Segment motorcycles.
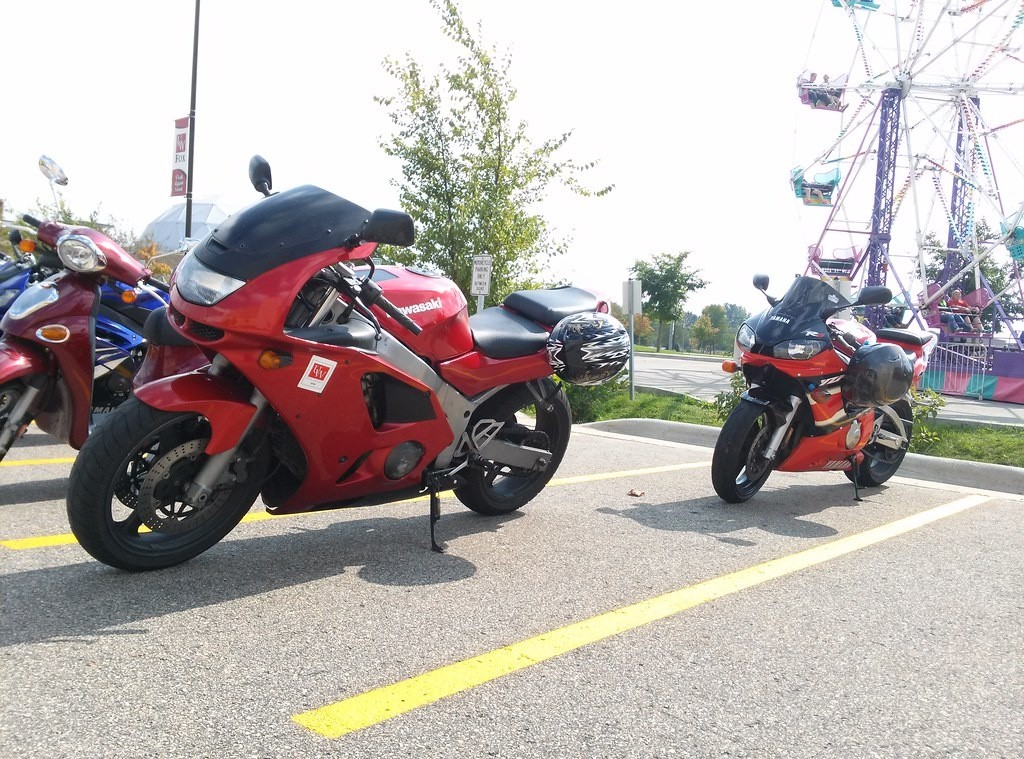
[0,155,202,465]
[67,154,631,574]
[710,273,942,505]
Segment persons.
[947,290,991,333]
[938,294,978,334]
[872,304,909,329]
[802,73,849,112]
[794,168,829,205]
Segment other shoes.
[969,328,977,333]
[983,329,991,333]
[822,201,829,204]
[806,198,812,204]
[953,328,963,333]
[816,98,850,111]
[896,324,908,329]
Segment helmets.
[546,312,631,386]
[842,344,914,406]
